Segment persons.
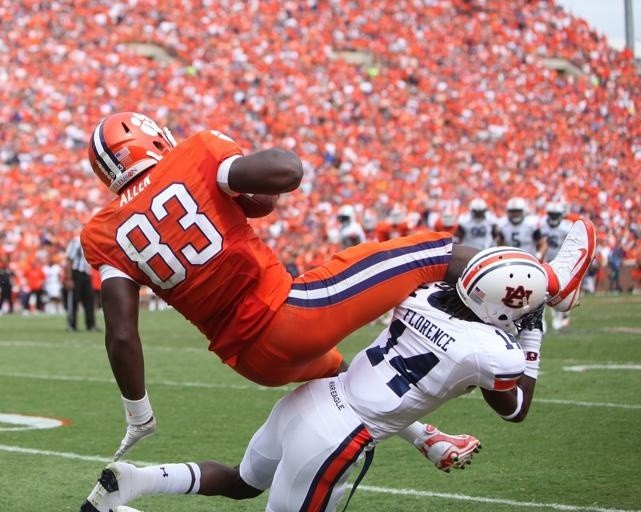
[79,245,549,512]
[81,112,596,473]
[1,0,640,330]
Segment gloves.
[111,420,158,460]
[514,311,544,347]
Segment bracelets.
[516,327,541,382]
[121,388,154,425]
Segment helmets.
[457,246,548,336]
[506,198,526,224]
[470,199,488,218]
[547,204,563,226]
[88,113,175,193]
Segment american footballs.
[235,193,279,218]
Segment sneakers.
[80,461,135,511]
[412,423,480,472]
[548,219,596,312]
